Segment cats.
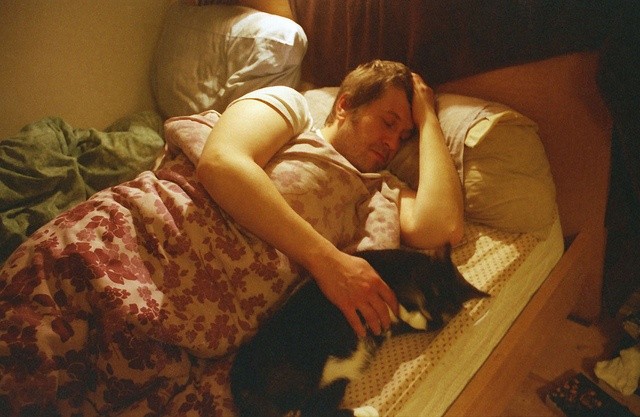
[230,241,492,417]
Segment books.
[544,372,640,417]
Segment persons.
[2,58,466,417]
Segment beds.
[1,51,612,417]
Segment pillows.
[149,1,307,118]
[293,88,556,233]
